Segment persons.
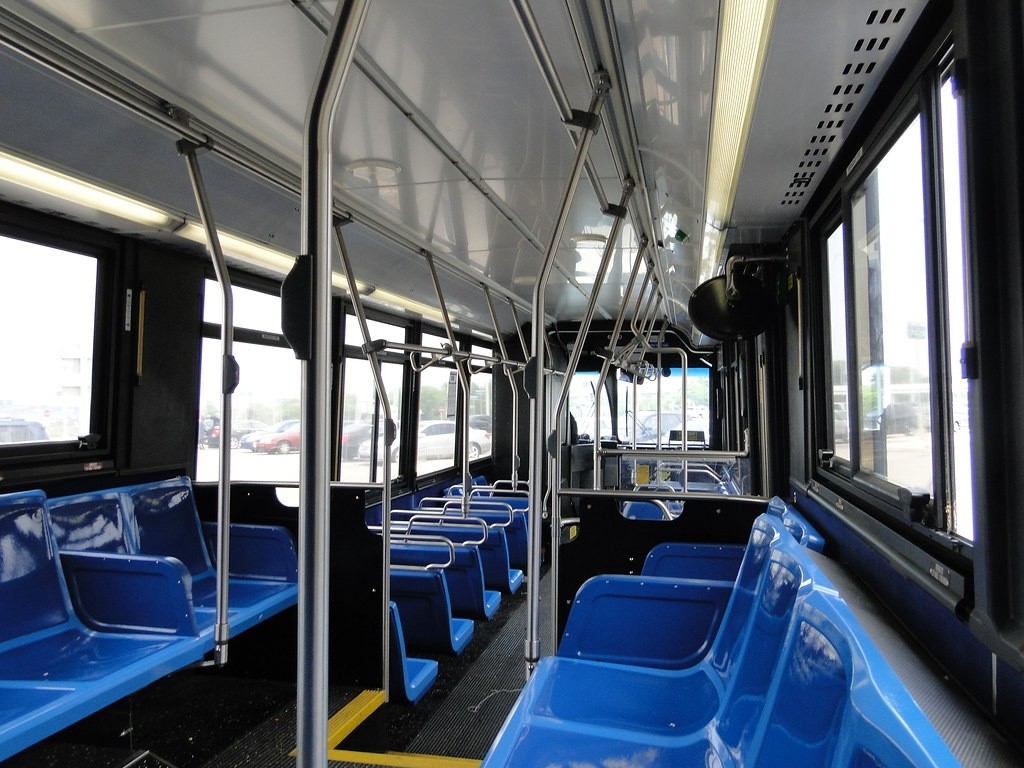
[619,368,630,382]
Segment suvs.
[0,417,50,445]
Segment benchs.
[0,462,1024,768]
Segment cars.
[619,406,704,446]
[198,413,492,464]
[834,397,971,443]
[378,419,492,464]
